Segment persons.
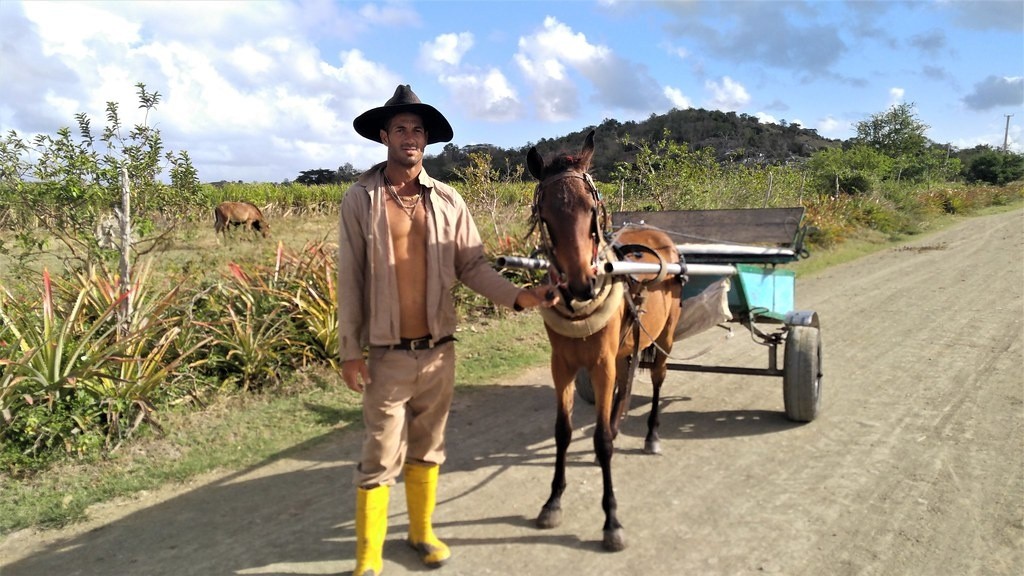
[337,85,561,576]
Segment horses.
[528,129,683,551]
[213,201,271,240]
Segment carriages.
[486,132,824,554]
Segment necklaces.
[383,169,426,221]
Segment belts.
[382,337,459,350]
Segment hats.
[353,85,453,145]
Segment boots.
[353,486,389,576]
[402,462,451,568]
